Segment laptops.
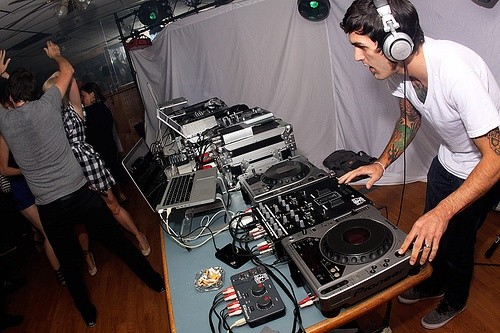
[157,109,217,139]
[122,137,217,214]
[146,82,187,110]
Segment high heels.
[86,253,97,276]
[138,234,151,256]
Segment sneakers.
[421,302,468,330]
[397,287,445,303]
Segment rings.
[424,243,431,248]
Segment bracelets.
[373,162,385,176]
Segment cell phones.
[215,244,253,269]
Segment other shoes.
[158,275,166,294]
[54,267,67,287]
[86,304,96,328]
[0,314,24,329]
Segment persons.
[79,81,127,203]
[1,71,151,277]
[0,39,165,328]
[1,91,68,286]
[336,0,500,329]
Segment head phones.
[372,0,415,62]
